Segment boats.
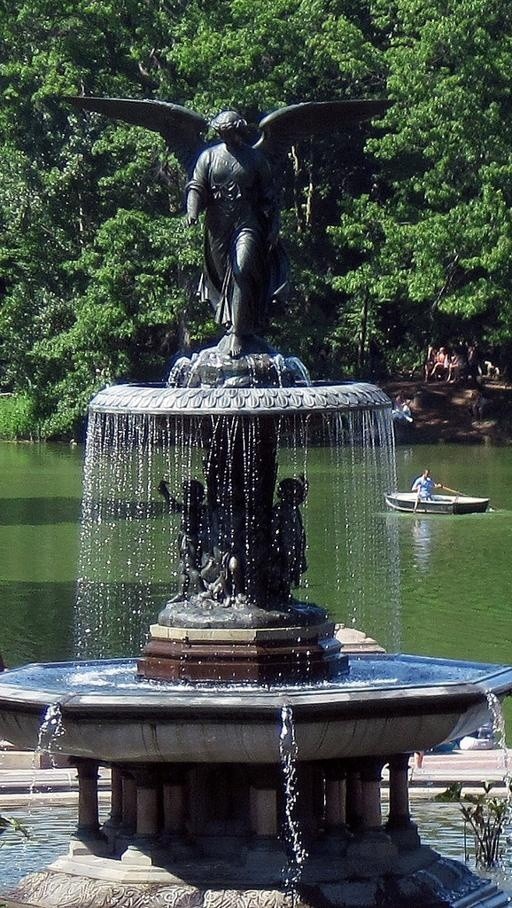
[387,510,509,523]
[384,491,490,516]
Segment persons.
[276,475,308,600]
[185,109,283,350]
[209,480,242,608]
[395,390,411,416]
[411,468,442,501]
[159,476,206,603]
[425,339,476,381]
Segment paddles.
[441,485,498,511]
[413,487,421,514]
